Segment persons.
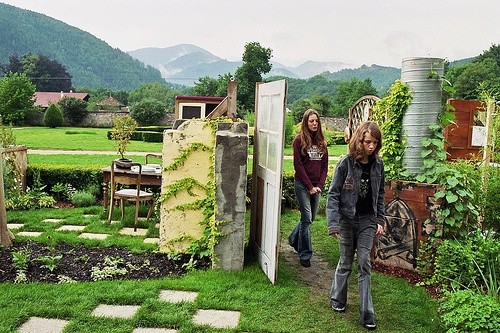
[325,122,386,329]
[287,109,329,268]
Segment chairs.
[143,152,163,208]
[106,159,156,231]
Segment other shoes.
[300,256,310,267]
[362,319,376,328]
[291,240,298,252]
[331,301,345,311]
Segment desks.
[100,165,162,215]
